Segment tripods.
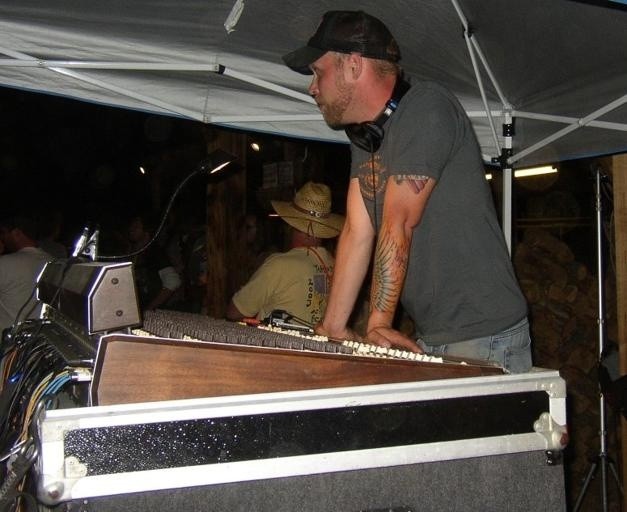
[568,170,624,512]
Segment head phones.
[345,77,412,153]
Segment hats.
[272,182,346,239]
[282,10,401,75]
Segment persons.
[224,180,347,332]
[280,11,534,374]
[0,204,281,335]
